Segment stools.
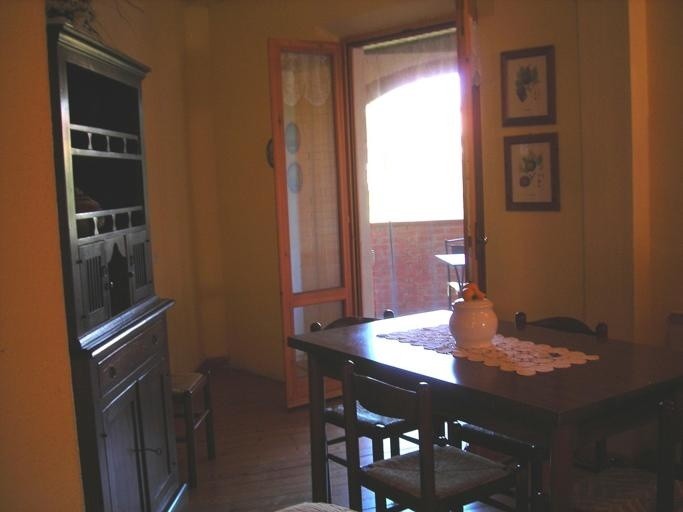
[171,372,215,489]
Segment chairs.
[631,310,682,510]
[448,312,606,512]
[341,358,522,511]
[445,238,466,310]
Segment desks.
[434,253,465,291]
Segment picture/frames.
[503,133,561,211]
[500,46,556,127]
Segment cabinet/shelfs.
[47,23,188,512]
